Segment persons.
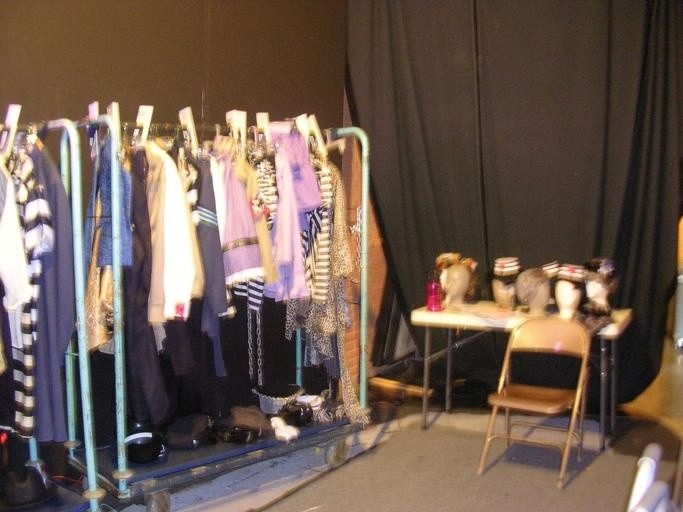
[434,251,621,324]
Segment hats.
[0,467,56,512]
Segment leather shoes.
[268,403,313,427]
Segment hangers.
[26,120,44,150]
[119,121,329,162]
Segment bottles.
[426,279,441,311]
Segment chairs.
[477,317,591,490]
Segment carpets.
[246,418,683,512]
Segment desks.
[411,301,634,452]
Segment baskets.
[252,383,304,414]
